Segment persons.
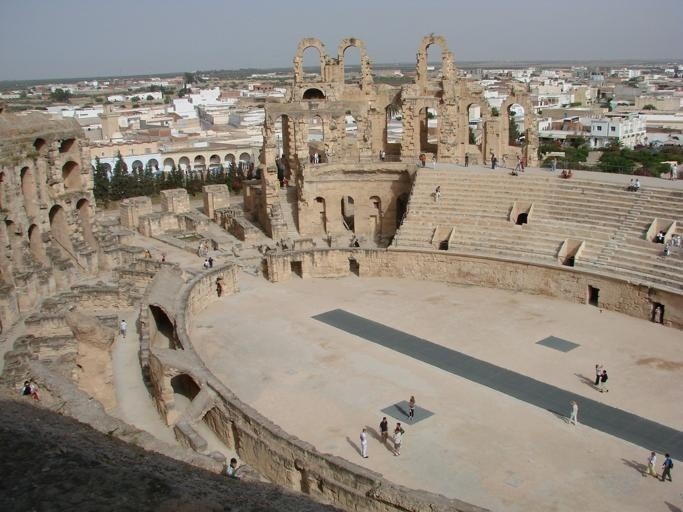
[627,178,634,192]
[431,158,436,169]
[656,230,682,257]
[119,319,128,339]
[281,177,288,188]
[314,151,319,163]
[381,151,386,161]
[654,307,662,323]
[24,380,39,400]
[670,163,678,183]
[419,154,427,167]
[379,417,388,443]
[566,399,579,427]
[644,451,660,478]
[311,152,316,164]
[593,363,604,386]
[391,429,402,457]
[225,458,241,481]
[408,396,416,420]
[408,399,415,413]
[435,186,442,201]
[20,381,30,396]
[633,178,641,192]
[144,241,214,270]
[395,422,405,449]
[359,428,369,458]
[464,153,470,167]
[598,369,609,392]
[491,153,573,179]
[659,454,674,482]
